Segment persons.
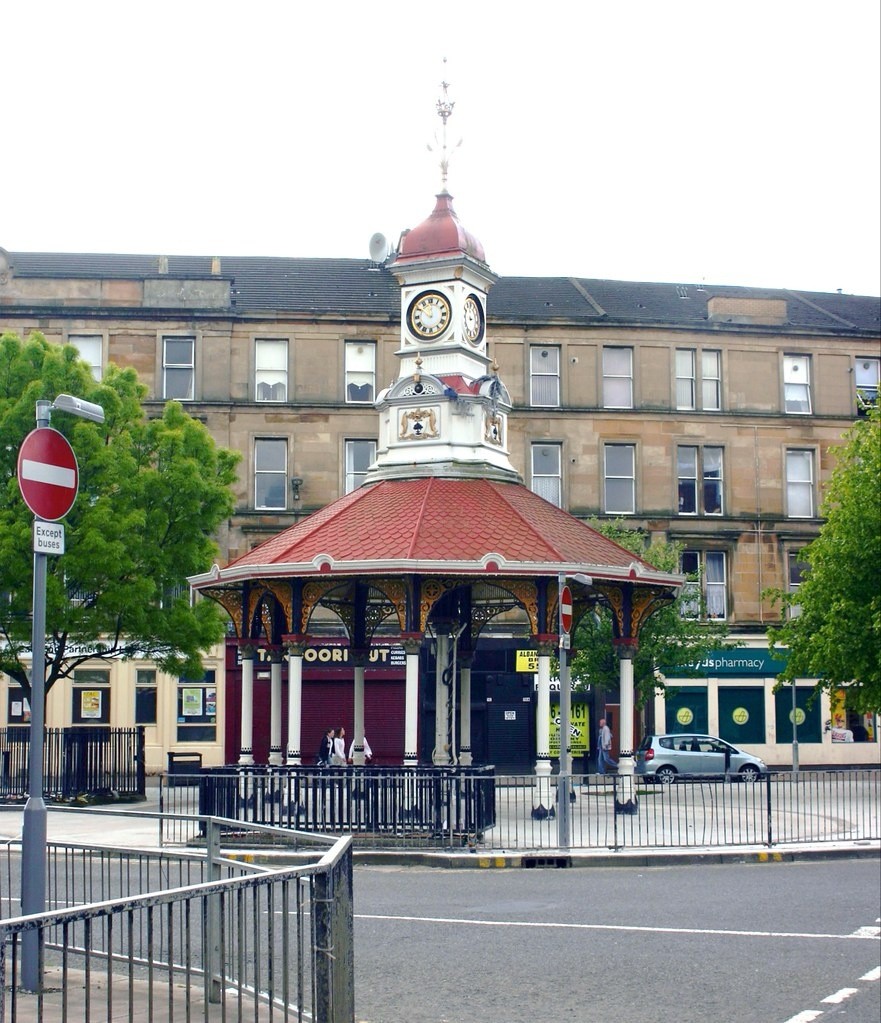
[318,726,335,765]
[597,718,618,775]
[348,728,372,763]
[334,726,347,765]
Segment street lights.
[557,569,593,852]
[22,393,106,993]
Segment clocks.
[410,295,449,337]
[464,300,482,340]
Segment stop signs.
[19,427,79,521]
[561,586,573,633]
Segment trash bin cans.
[167,750,203,786]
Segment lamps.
[292,477,303,500]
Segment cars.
[635,735,769,784]
